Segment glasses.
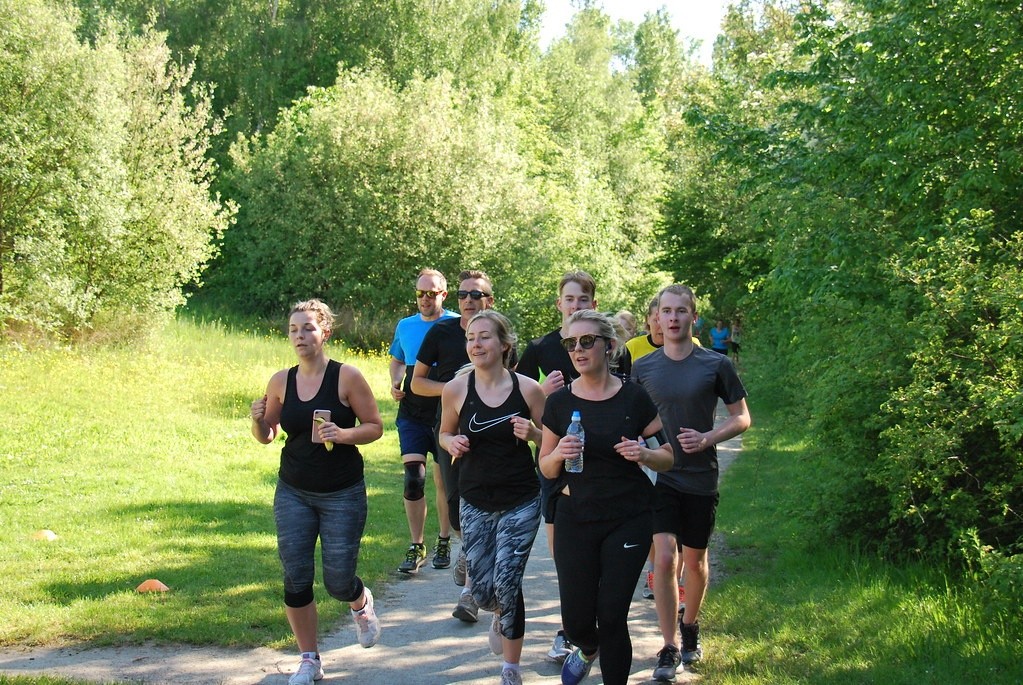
[415,289,444,298]
[457,291,490,300]
[560,334,606,352]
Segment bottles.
[565,411,585,473]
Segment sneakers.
[643,572,654,599]
[431,534,452,569]
[679,614,702,663]
[289,652,323,685]
[678,582,687,613]
[562,648,599,685]
[652,645,684,683]
[501,669,522,685]
[452,548,466,586]
[351,586,381,649]
[396,546,428,574]
[489,613,503,655]
[548,631,574,661]
[452,588,478,623]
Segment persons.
[538,311,674,685]
[632,284,751,682]
[709,319,731,354]
[439,309,545,685]
[692,318,704,339]
[627,297,701,612]
[730,319,742,363]
[251,301,383,685]
[515,269,633,662]
[389,270,462,574]
[614,309,636,337]
[411,271,519,622]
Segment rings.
[631,452,633,455]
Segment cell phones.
[312,410,331,444]
